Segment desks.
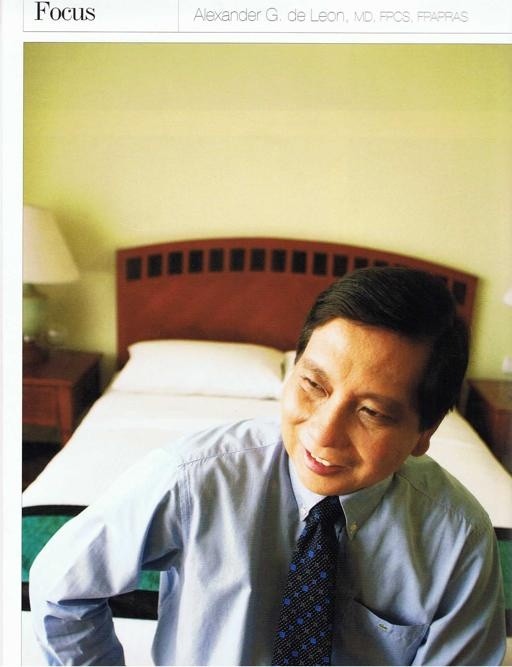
[23,345,101,453]
[464,375,512,474]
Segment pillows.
[114,338,287,398]
[281,349,297,379]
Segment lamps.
[23,203,84,357]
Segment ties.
[272,497,345,665]
